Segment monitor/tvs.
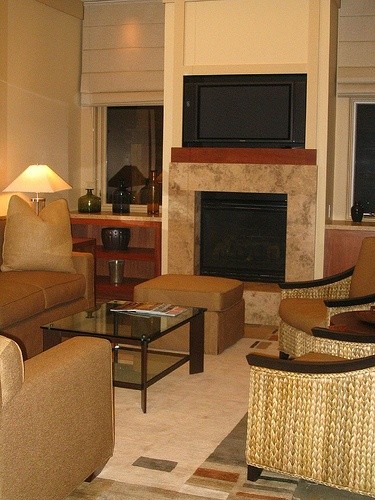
[182,74,307,150]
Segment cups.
[108,258,125,284]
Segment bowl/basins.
[101,228,130,251]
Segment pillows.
[0,195,78,275]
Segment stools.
[134,274,245,355]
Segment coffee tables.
[40,299,207,413]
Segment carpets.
[65,323,301,500]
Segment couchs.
[0,216,95,362]
[277,237,375,359]
[245,327,375,500]
[0,335,115,500]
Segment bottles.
[113,176,130,214]
[78,188,101,214]
[147,170,159,217]
[351,201,363,222]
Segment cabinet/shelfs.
[70,210,161,305]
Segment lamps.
[2,163,73,215]
